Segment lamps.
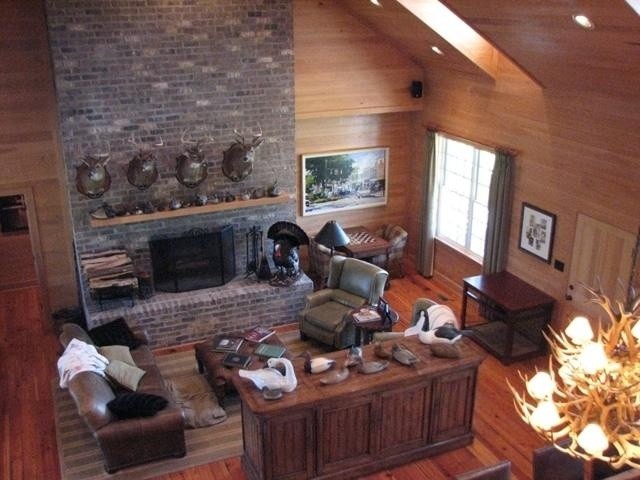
[314,221,352,257]
[505,276,640,471]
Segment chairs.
[298,255,389,351]
[369,223,408,291]
[372,298,454,343]
[583,438,640,480]
[533,438,583,479]
[455,459,512,479]
[308,235,347,291]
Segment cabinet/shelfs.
[231,329,486,480]
[461,271,557,366]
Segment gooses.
[238,357,297,392]
[416,309,462,344]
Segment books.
[245,359,254,368]
[351,308,381,327]
[211,334,245,354]
[238,325,276,344]
[253,343,286,359]
[223,351,251,370]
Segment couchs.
[58,322,186,474]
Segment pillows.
[106,392,169,418]
[99,345,136,366]
[102,359,146,392]
[88,317,140,351]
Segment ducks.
[431,340,468,359]
[294,340,419,384]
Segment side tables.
[351,306,400,347]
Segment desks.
[342,225,393,261]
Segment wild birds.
[267,221,309,280]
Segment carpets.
[50,321,338,480]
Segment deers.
[176,128,214,188]
[127,131,164,190]
[221,122,264,181]
[76,141,111,199]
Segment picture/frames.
[518,202,556,265]
[300,144,390,218]
